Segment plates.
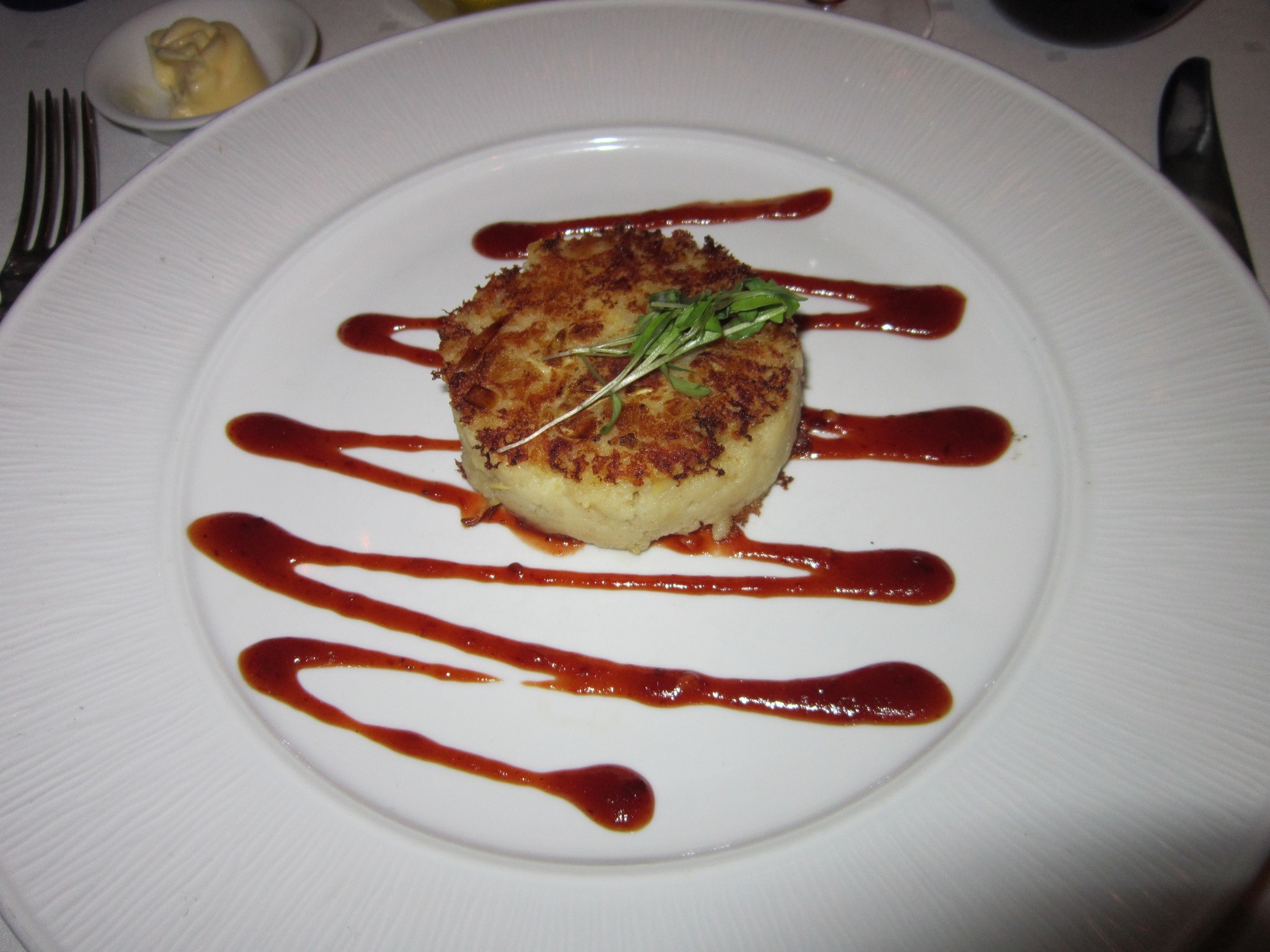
[1,0,1270,952]
[83,0,319,146]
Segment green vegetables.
[496,277,810,455]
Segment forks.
[0,88,95,317]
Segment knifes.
[1157,56,1256,278]
